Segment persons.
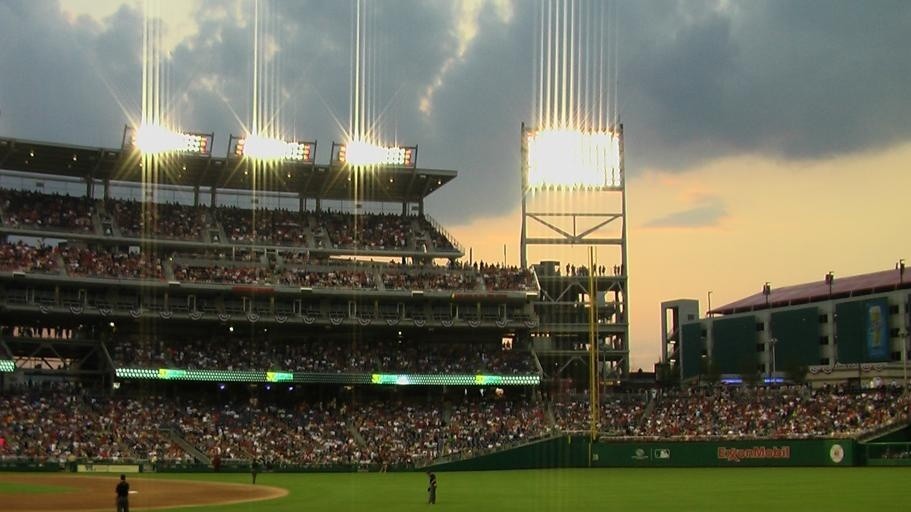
[425,470,437,504]
[249,457,258,484]
[565,263,624,276]
[0,234,536,292]
[0,184,456,253]
[2,383,909,472]
[104,321,542,377]
[115,473,130,512]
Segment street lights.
[225,132,317,210]
[521,122,628,277]
[327,141,419,216]
[117,125,214,207]
